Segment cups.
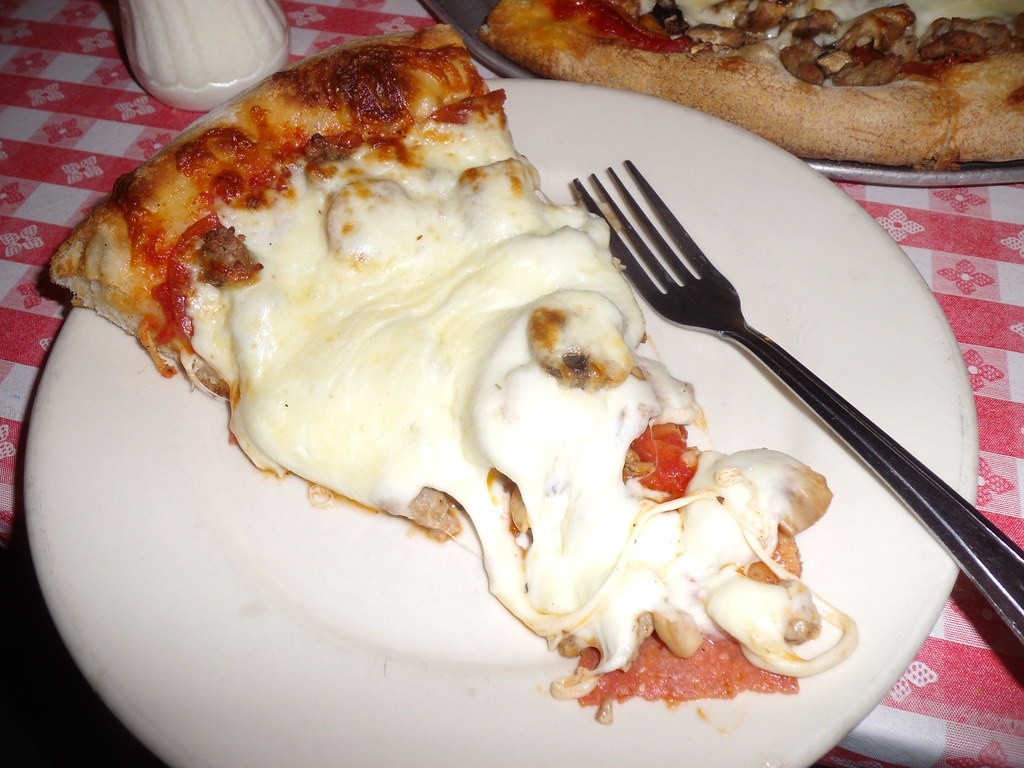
[115,1,290,107]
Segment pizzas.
[50,22,858,696]
[477,0,1022,165]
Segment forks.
[571,158,1024,649]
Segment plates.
[23,78,977,768]
